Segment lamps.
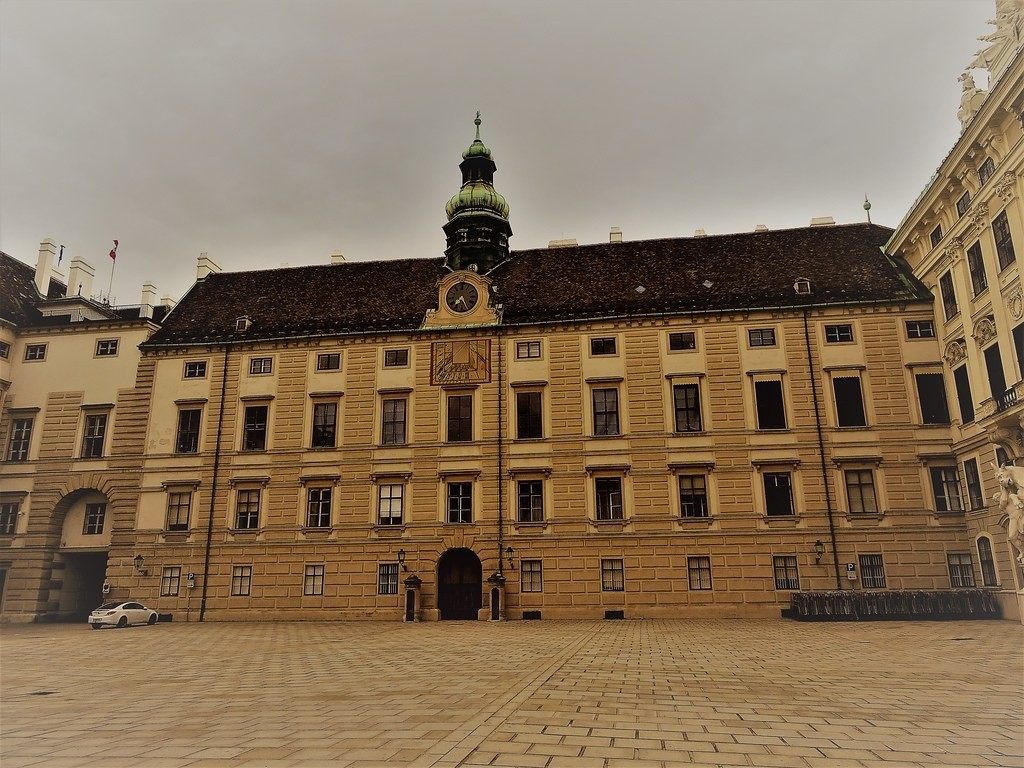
[814,539,825,561]
[398,549,407,570]
[133,554,148,576]
[505,547,514,568]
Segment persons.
[992,492,1024,562]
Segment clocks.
[446,280,480,313]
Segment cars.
[88,601,159,629]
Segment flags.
[110,239,118,260]
